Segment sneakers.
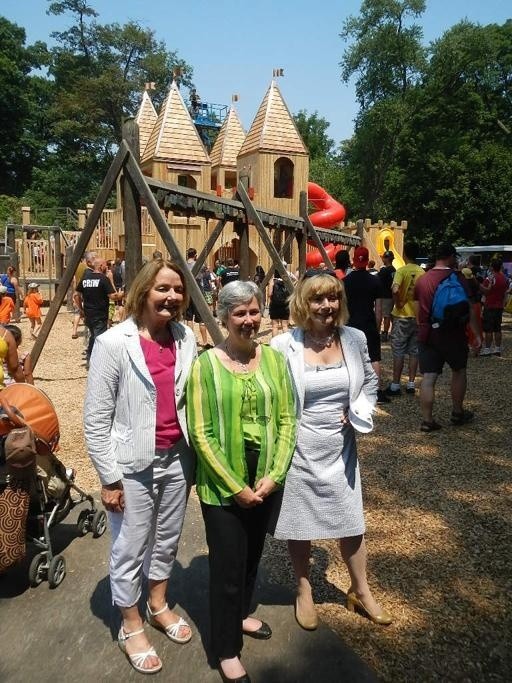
[203,343,214,349]
[381,333,392,344]
[30,327,38,340]
[72,334,78,338]
[470,348,501,360]
[376,383,415,404]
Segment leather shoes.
[242,616,272,639]
[215,656,252,683]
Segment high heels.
[347,587,393,624]
[295,594,318,629]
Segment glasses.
[303,268,336,280]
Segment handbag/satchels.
[202,289,214,305]
[503,292,512,313]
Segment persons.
[153,251,162,259]
[333,240,509,432]
[0,228,61,486]
[72,248,126,368]
[269,268,392,630]
[82,258,198,673]
[184,248,239,350]
[254,261,298,337]
[185,280,298,682]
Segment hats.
[5,425,37,481]
[380,251,394,259]
[335,250,351,269]
[352,246,368,267]
[434,242,461,256]
[27,283,40,289]
[461,268,473,279]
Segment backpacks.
[273,280,287,308]
[429,273,470,331]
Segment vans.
[453,245,512,276]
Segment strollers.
[1,382,107,589]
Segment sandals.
[420,418,442,431]
[146,600,193,643]
[117,621,162,675]
[449,408,476,425]
